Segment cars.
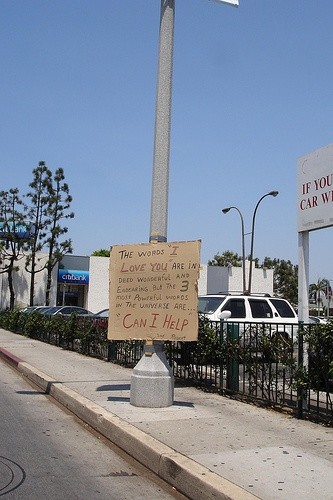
[16,306,109,341]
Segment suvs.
[164,292,299,365]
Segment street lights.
[222,191,279,295]
